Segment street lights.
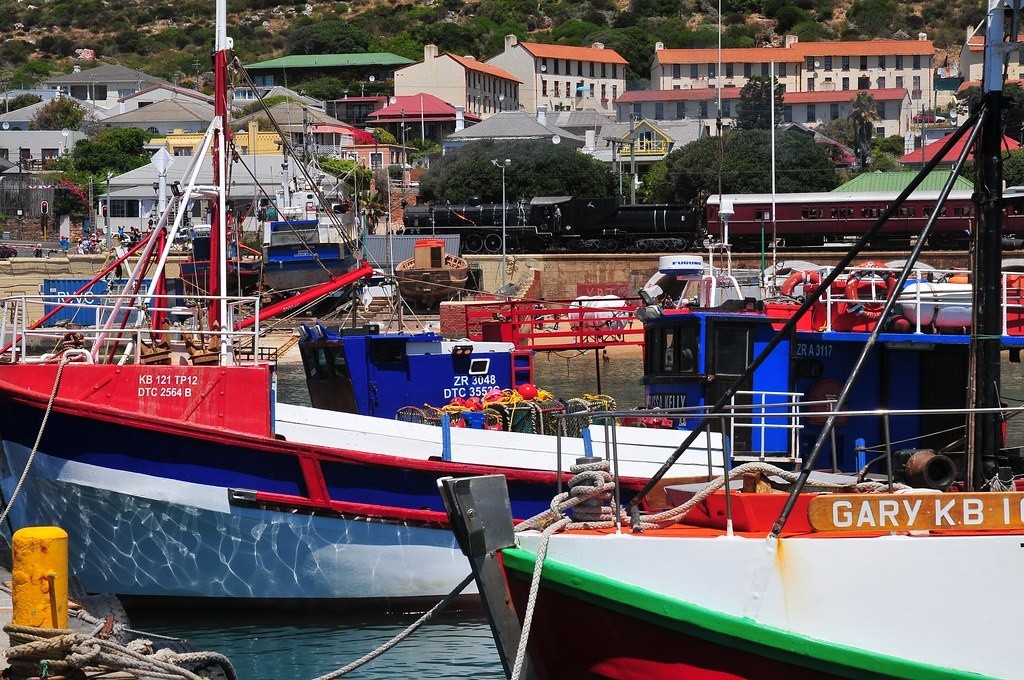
[107,171,115,251]
[491,158,512,291]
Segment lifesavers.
[781,271,828,311]
[845,260,898,318]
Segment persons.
[0,218,198,258]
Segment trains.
[396,183,1024,256]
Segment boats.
[392,235,469,309]
[0,0,949,630]
[433,0,1024,680]
[261,136,362,295]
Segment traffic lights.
[41,200,48,214]
[103,204,108,218]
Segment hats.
[78,239,81,241]
[60,235,65,239]
[37,244,42,247]
[85,224,194,245]
[80,235,83,238]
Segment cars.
[0,245,18,258]
